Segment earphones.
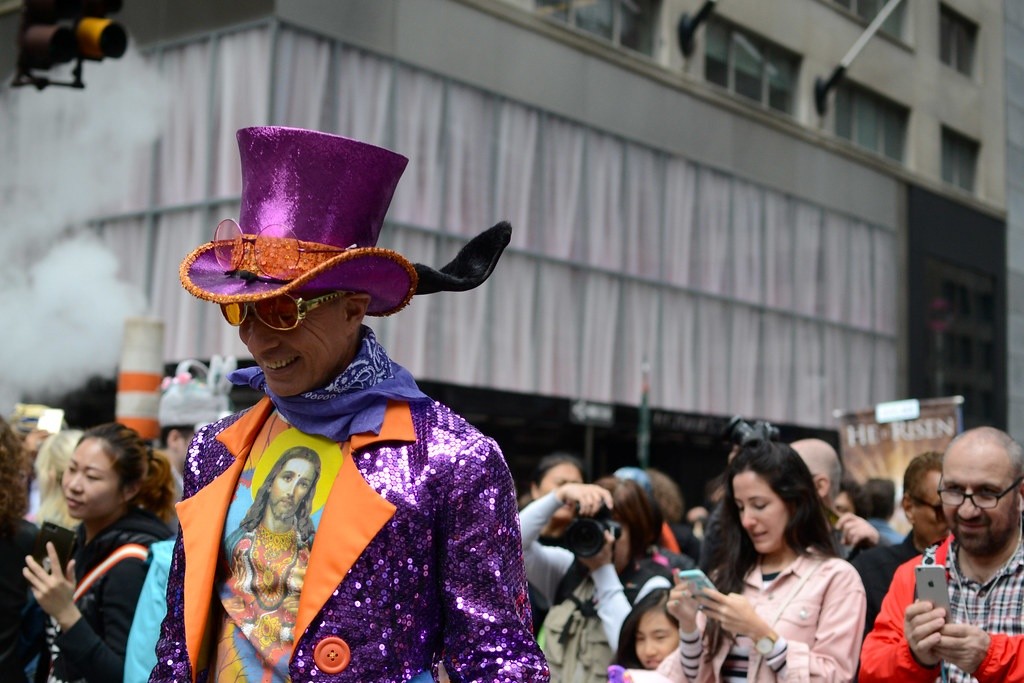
[1017,493,1024,512]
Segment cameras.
[722,414,779,448]
[562,503,621,559]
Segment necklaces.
[948,536,1021,624]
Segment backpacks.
[553,548,697,607]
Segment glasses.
[937,475,1024,510]
[211,218,346,280]
[219,290,356,331]
[909,494,945,523]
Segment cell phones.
[679,569,717,599]
[34,520,76,576]
[915,564,953,624]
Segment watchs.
[755,631,779,655]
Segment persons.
[0,380,229,683]
[858,427,1024,683]
[518,444,741,683]
[665,439,867,683]
[790,439,944,683]
[146,238,551,683]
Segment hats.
[178,125,512,318]
[157,354,237,429]
